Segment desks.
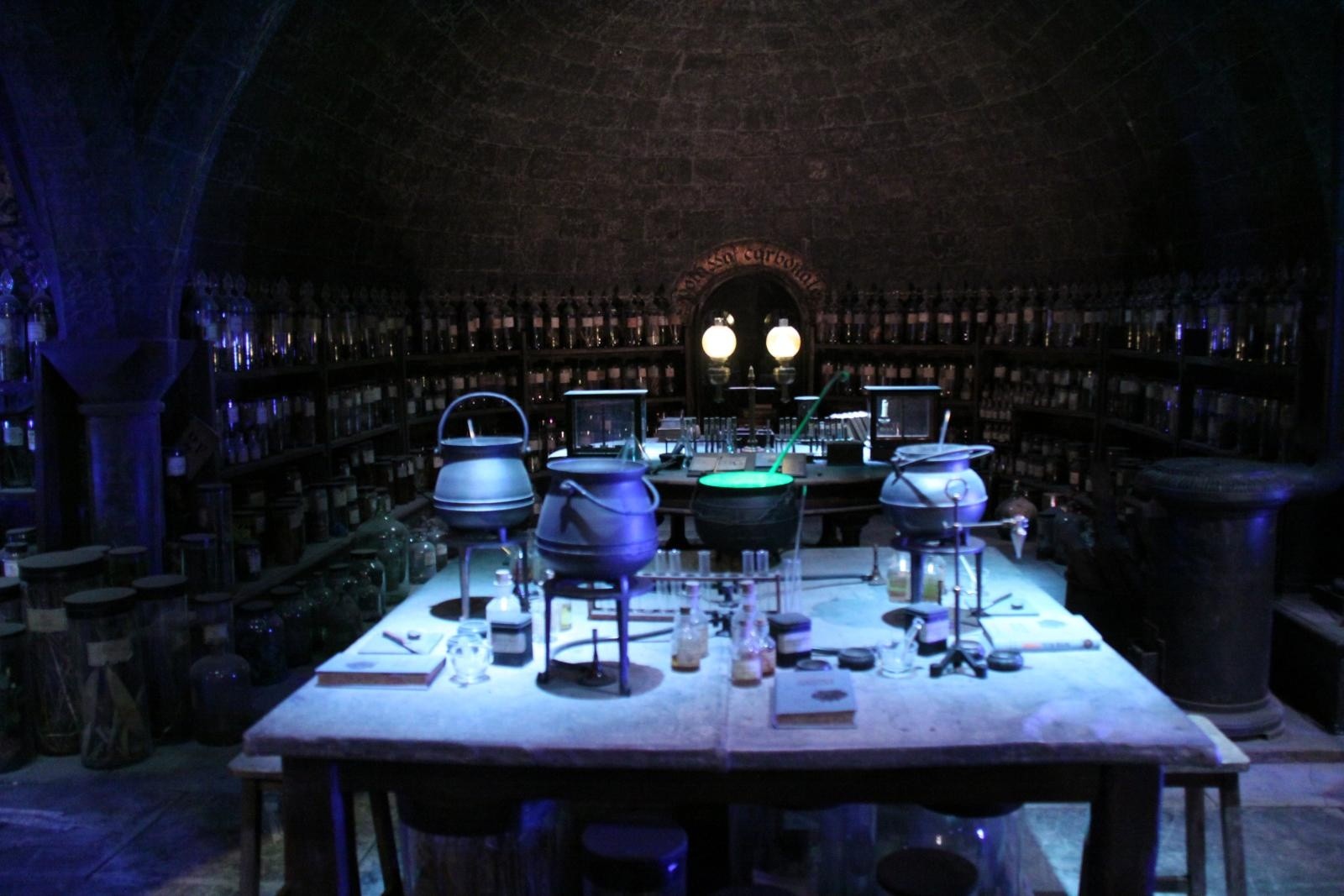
[242,509,1218,896]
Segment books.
[980,615,1103,652]
[687,456,746,475]
[773,670,858,728]
[314,652,447,689]
[755,453,782,472]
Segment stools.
[1151,714,1253,896]
[226,749,283,896]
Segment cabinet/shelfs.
[0,143,1344,740]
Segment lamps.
[765,316,802,403]
[700,315,739,406]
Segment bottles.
[399,795,1023,896]
[0,270,681,746]
[994,479,1038,544]
[815,265,1305,495]
[670,580,775,688]
[887,548,945,602]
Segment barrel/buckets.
[878,443,995,540]
[539,457,659,571]
[432,390,536,528]
